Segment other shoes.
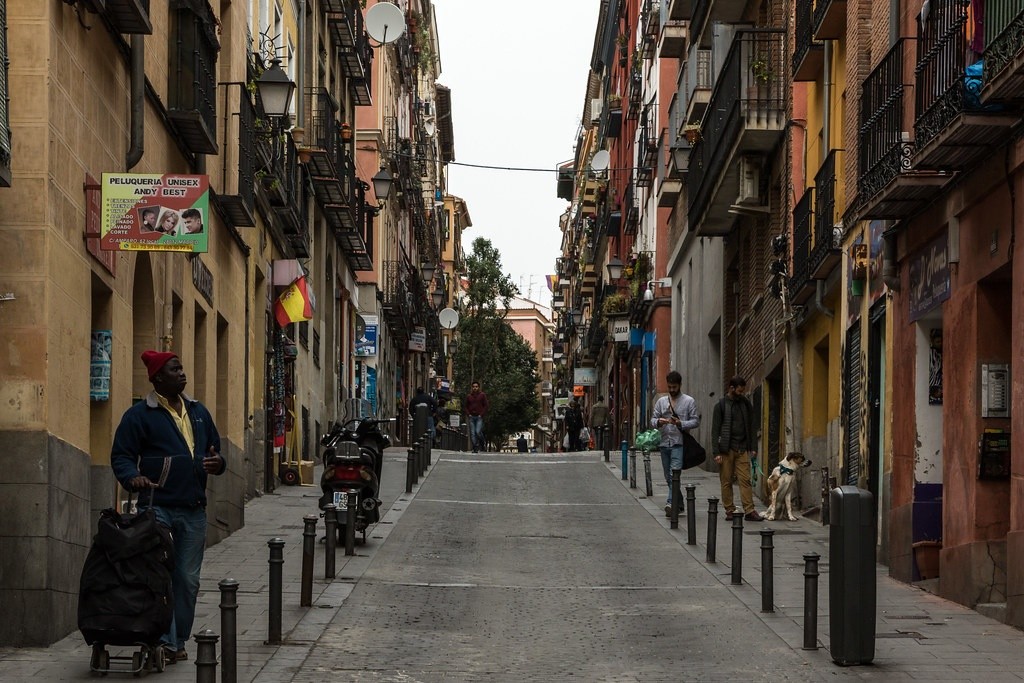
[678,507,685,514]
[176,648,187,660]
[664,504,672,517]
[745,511,764,521]
[725,511,733,521]
[474,448,478,453]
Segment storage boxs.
[280,460,314,485]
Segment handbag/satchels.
[587,428,595,449]
[562,432,570,448]
[579,426,590,443]
[435,420,447,430]
[681,432,706,470]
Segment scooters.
[318,415,399,546]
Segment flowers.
[340,122,349,129]
[749,57,778,87]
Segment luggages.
[77,482,177,676]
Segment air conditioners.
[589,98,603,125]
[735,153,764,206]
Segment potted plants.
[297,144,314,164]
[614,33,628,57]
[606,93,624,108]
[910,531,942,580]
[604,294,632,311]
[675,118,706,152]
[409,21,427,34]
[404,8,423,26]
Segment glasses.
[472,386,479,388]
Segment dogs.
[758,452,812,520]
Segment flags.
[274,276,312,327]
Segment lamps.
[364,166,394,212]
[248,58,298,139]
[570,305,591,329]
[606,253,626,282]
[420,258,436,289]
[430,285,444,317]
[445,339,459,359]
[643,277,672,301]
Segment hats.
[141,350,179,382]
[597,395,604,401]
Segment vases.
[619,59,627,68]
[339,130,352,139]
[744,86,773,110]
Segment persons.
[110,351,226,664]
[517,435,528,453]
[466,382,488,453]
[712,377,763,521]
[410,387,437,437]
[433,399,450,430]
[565,395,610,451]
[651,371,699,516]
[140,209,203,237]
